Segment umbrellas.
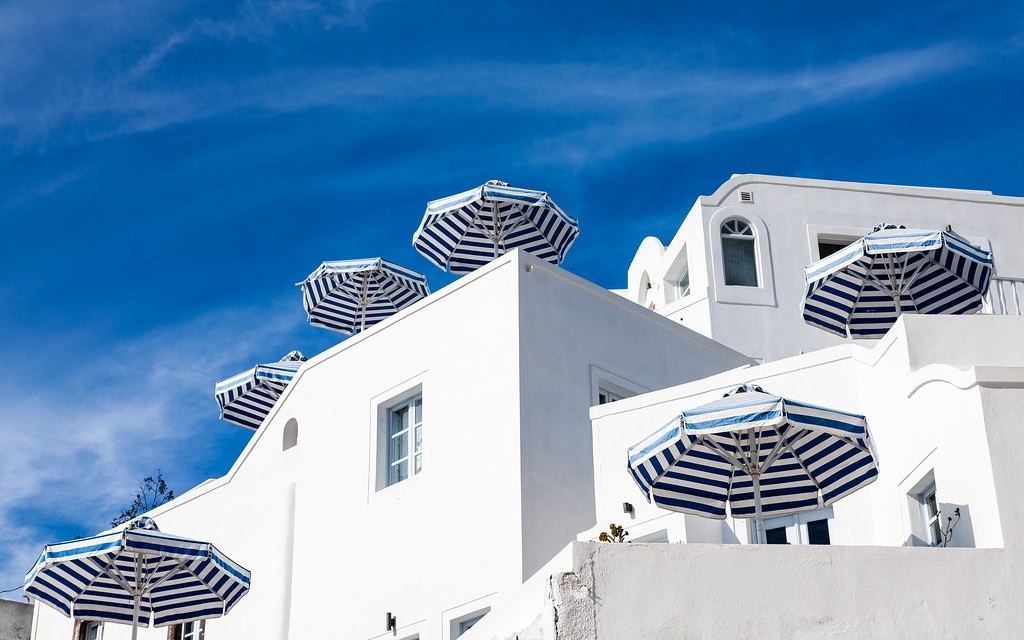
[215,350,308,431]
[24,516,251,640]
[412,180,580,275]
[799,223,994,339]
[627,384,880,544]
[295,256,431,336]
